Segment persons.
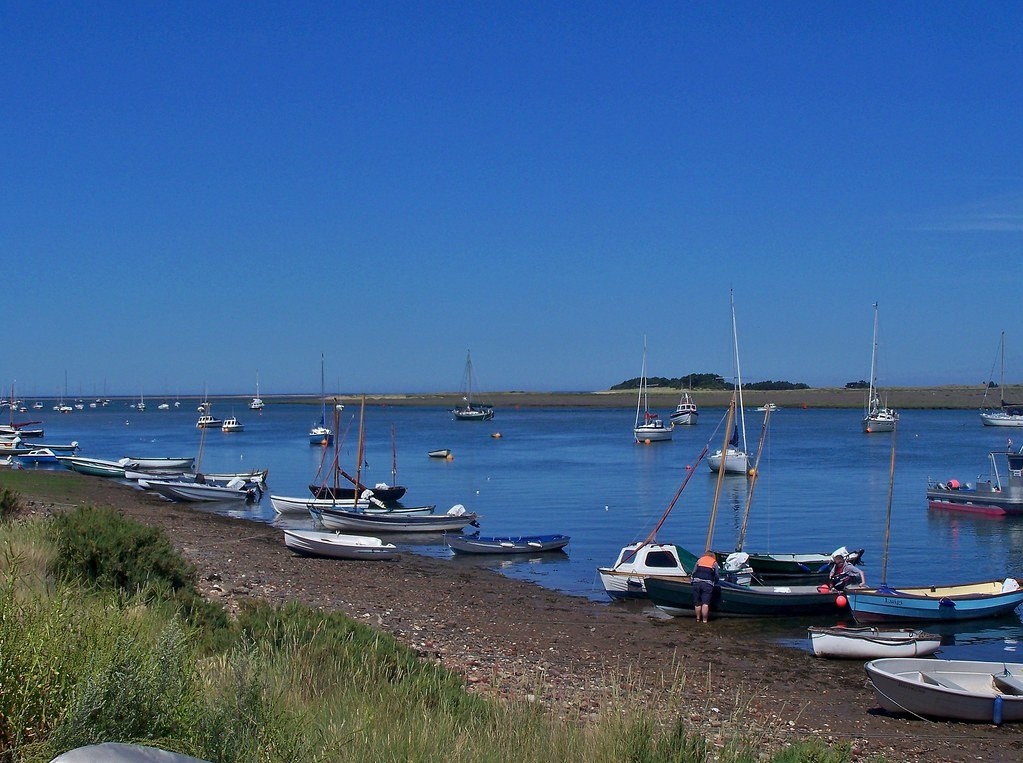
[690,551,719,624]
[828,555,865,588]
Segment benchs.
[992,672,1023,696]
[920,669,969,695]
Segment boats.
[18,449,75,464]
[0,369,112,454]
[220,416,246,433]
[278,527,400,561]
[20,441,79,453]
[756,402,777,413]
[0,455,16,467]
[55,453,269,503]
[862,655,1023,725]
[923,440,1023,518]
[441,530,572,556]
[640,570,850,619]
[805,625,942,660]
[670,391,698,426]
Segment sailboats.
[307,352,334,445]
[840,412,1023,627]
[709,289,753,474]
[860,301,898,434]
[248,368,265,410]
[131,381,222,429]
[450,349,498,421]
[590,373,867,602]
[976,330,1023,428]
[631,332,674,444]
[264,384,481,534]
